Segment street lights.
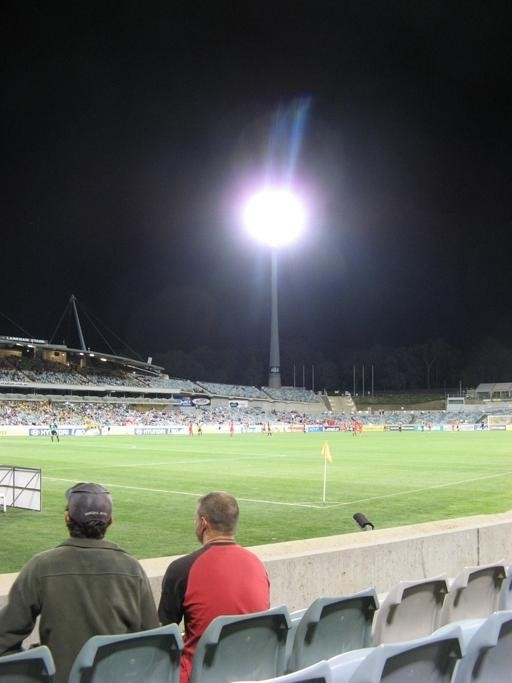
[242,184,308,388]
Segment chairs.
[0,561,512,683]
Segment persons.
[0,356,321,403]
[1,483,158,683]
[159,491,270,683]
[50,418,59,444]
[1,401,512,438]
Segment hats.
[65,482,113,525]
